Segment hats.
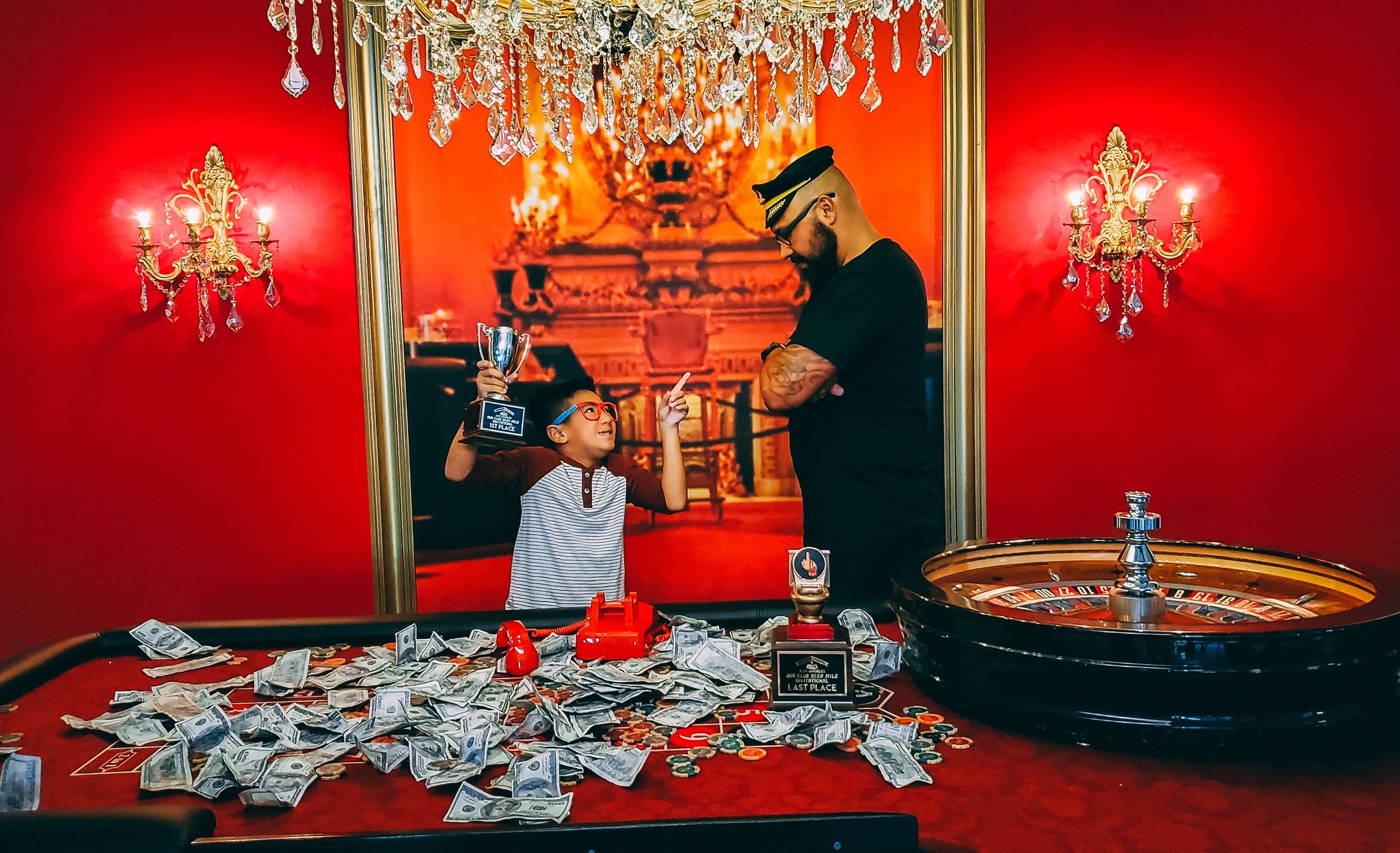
[751,146,835,229]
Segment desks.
[2,596,1400,853]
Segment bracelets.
[760,340,785,363]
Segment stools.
[628,306,728,526]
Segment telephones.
[497,591,671,678]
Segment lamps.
[262,1,953,169]
[123,147,285,341]
[1061,124,1205,341]
[509,40,821,253]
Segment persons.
[748,142,932,598]
[443,361,697,610]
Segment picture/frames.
[333,1,988,617]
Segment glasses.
[772,192,836,246]
[551,401,619,426]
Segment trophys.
[458,320,534,454]
[768,546,856,710]
